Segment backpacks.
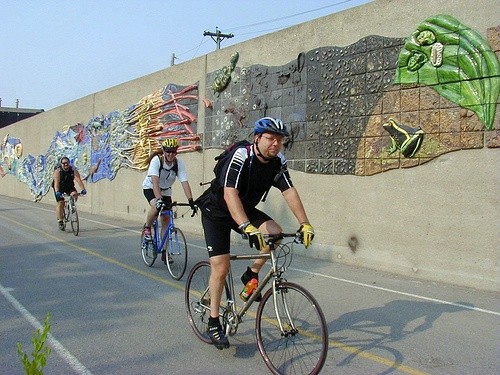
[213,140,285,202]
[148,151,179,178]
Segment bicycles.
[61,193,82,236]
[141,202,197,280]
[185,231,328,375]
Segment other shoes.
[68,204,75,214]
[207,322,230,349]
[241,266,262,302]
[58,220,65,231]
[143,223,152,240]
[162,251,174,263]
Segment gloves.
[188,198,198,210]
[55,191,62,201]
[156,199,166,216]
[297,222,315,249]
[242,224,266,251]
[81,190,86,194]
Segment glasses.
[262,136,284,144]
[164,150,176,153]
[62,162,69,164]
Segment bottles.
[239,278,258,302]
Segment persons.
[201,117,314,349]
[51,157,86,231]
[142,138,194,264]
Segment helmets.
[162,138,179,149]
[254,116,289,136]
